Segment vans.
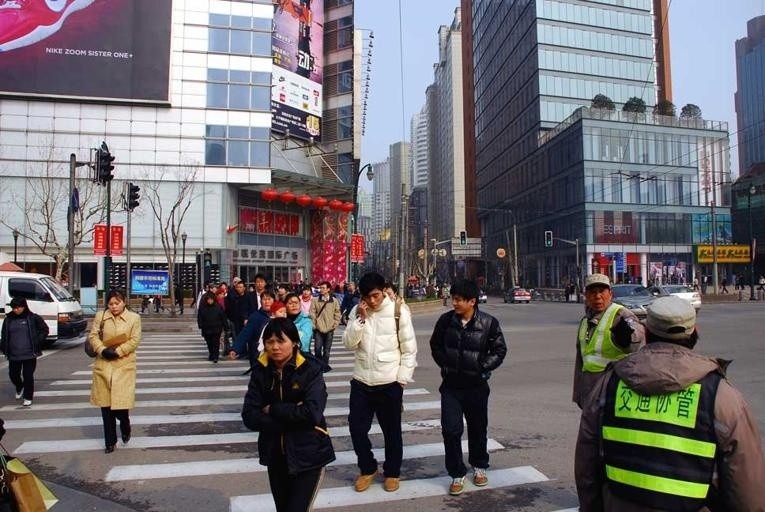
[0,271,86,346]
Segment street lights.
[353,163,375,285]
[746,179,758,301]
[180,231,187,297]
[10,227,22,263]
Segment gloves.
[102,348,118,359]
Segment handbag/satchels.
[84,343,98,357]
[3,455,59,512]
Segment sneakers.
[448,473,468,494]
[471,465,487,486]
[15,388,24,399]
[355,469,378,490]
[23,399,32,405]
[104,445,114,453]
[384,477,400,490]
[122,433,131,443]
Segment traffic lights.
[203,252,212,267]
[544,231,553,247]
[96,151,114,185]
[129,182,140,211]
[460,231,466,245]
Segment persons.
[0,294,50,406]
[342,273,417,491]
[241,317,336,512]
[191,274,341,371]
[429,276,508,496]
[334,279,449,321]
[693,270,765,295]
[575,285,580,303]
[173,283,184,315]
[140,295,164,313]
[564,285,572,303]
[86,288,147,455]
[573,294,765,511]
[572,273,646,410]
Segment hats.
[585,274,610,288]
[645,295,696,339]
[270,302,284,313]
[6,298,22,306]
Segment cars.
[504,286,530,304]
[477,287,488,303]
[607,283,653,322]
[648,284,701,310]
